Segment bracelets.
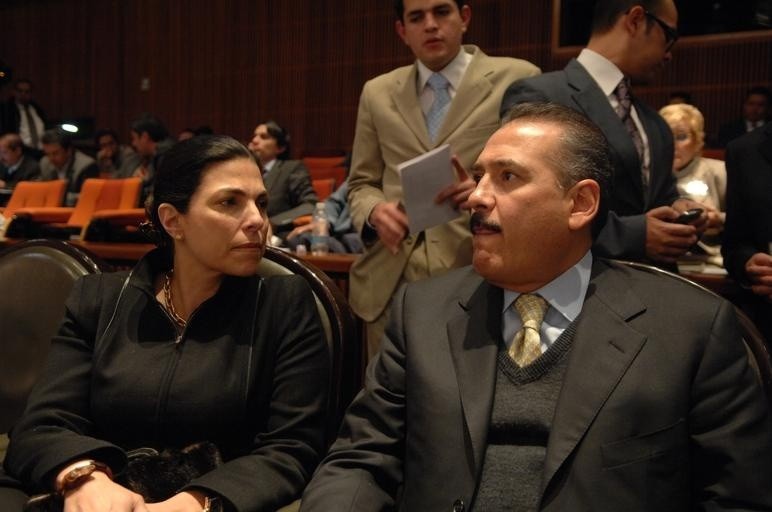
[55,460,115,501]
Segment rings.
[748,265,753,275]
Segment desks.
[297,252,367,273]
[87,244,159,261]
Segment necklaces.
[163,261,192,330]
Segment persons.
[34,127,99,207]
[664,89,692,106]
[498,2,723,277]
[3,132,329,512]
[111,113,180,207]
[4,133,40,189]
[245,121,316,232]
[95,128,135,179]
[716,118,771,356]
[271,176,365,251]
[652,100,730,265]
[346,0,546,367]
[298,99,770,511]
[179,128,194,141]
[727,84,770,141]
[3,78,51,160]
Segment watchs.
[199,486,225,512]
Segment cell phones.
[673,207,704,224]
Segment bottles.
[311,202,330,257]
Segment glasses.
[626,5,680,50]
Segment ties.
[505,290,551,376]
[610,71,648,205]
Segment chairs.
[611,261,767,508]
[304,156,348,201]
[4,241,104,441]
[2,171,151,244]
[257,244,345,507]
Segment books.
[395,141,466,235]
